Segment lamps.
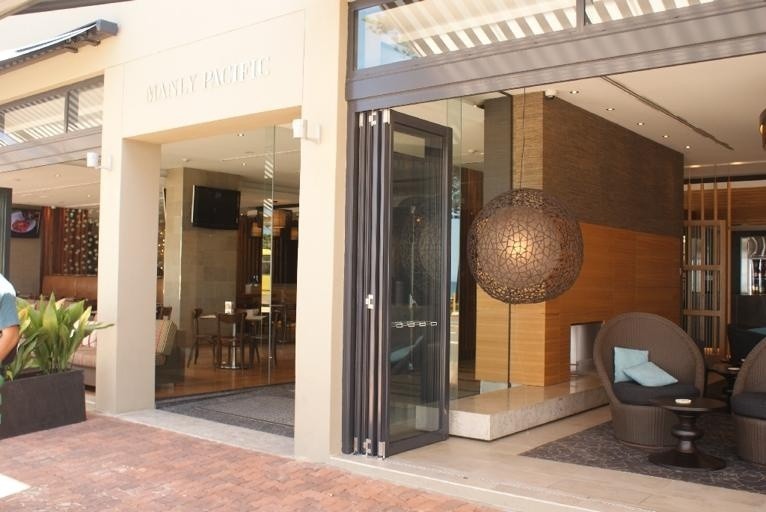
[465,87,586,306]
[85,150,113,174]
[292,119,322,146]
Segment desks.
[236,293,277,308]
[647,395,729,473]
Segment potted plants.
[0,291,117,442]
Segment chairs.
[160,303,295,374]
[591,308,766,466]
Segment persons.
[0,273,20,363]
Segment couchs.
[23,298,188,390]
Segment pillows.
[69,318,99,349]
